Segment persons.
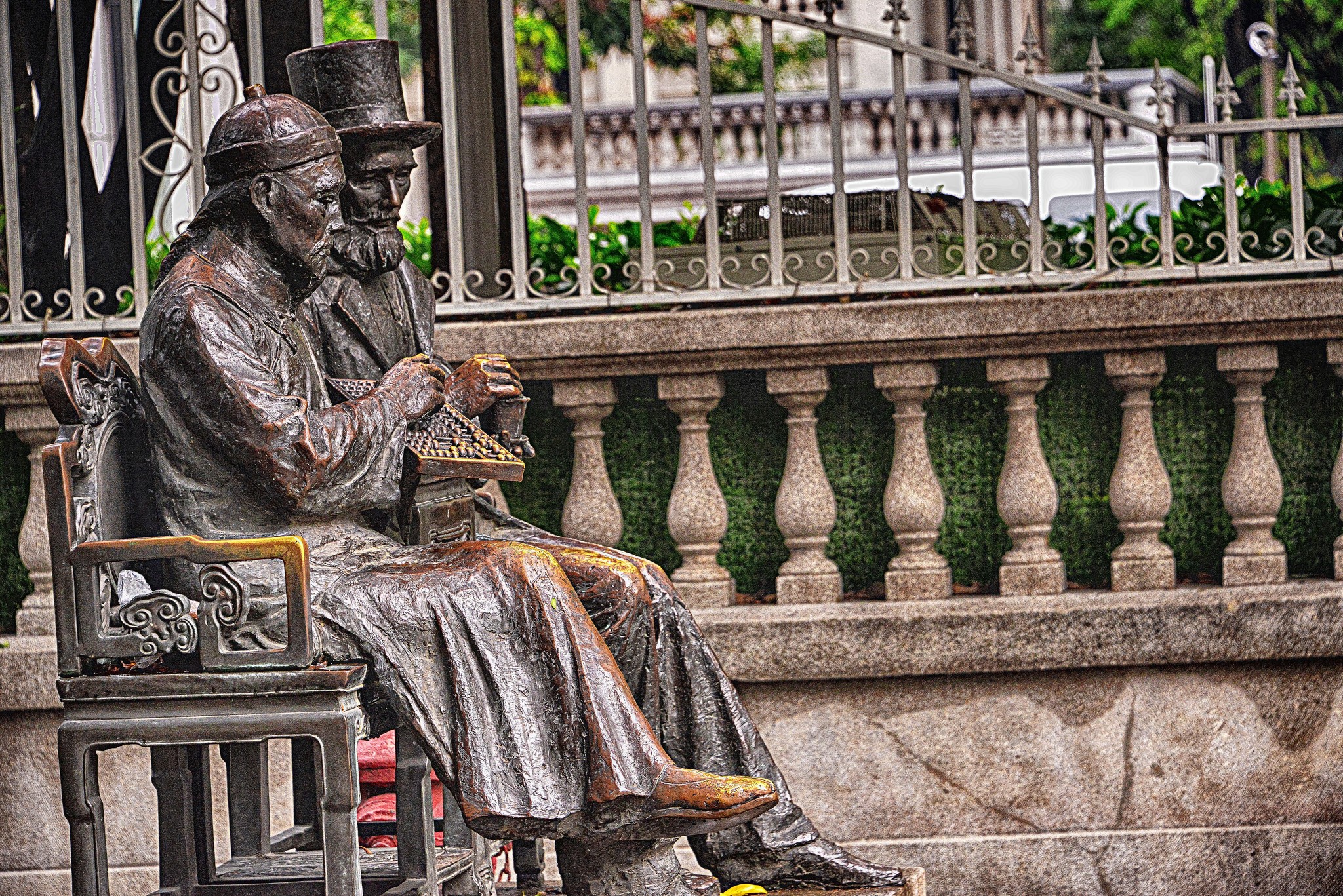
[284,38,908,895]
[137,85,781,837]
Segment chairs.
[35,335,502,896]
